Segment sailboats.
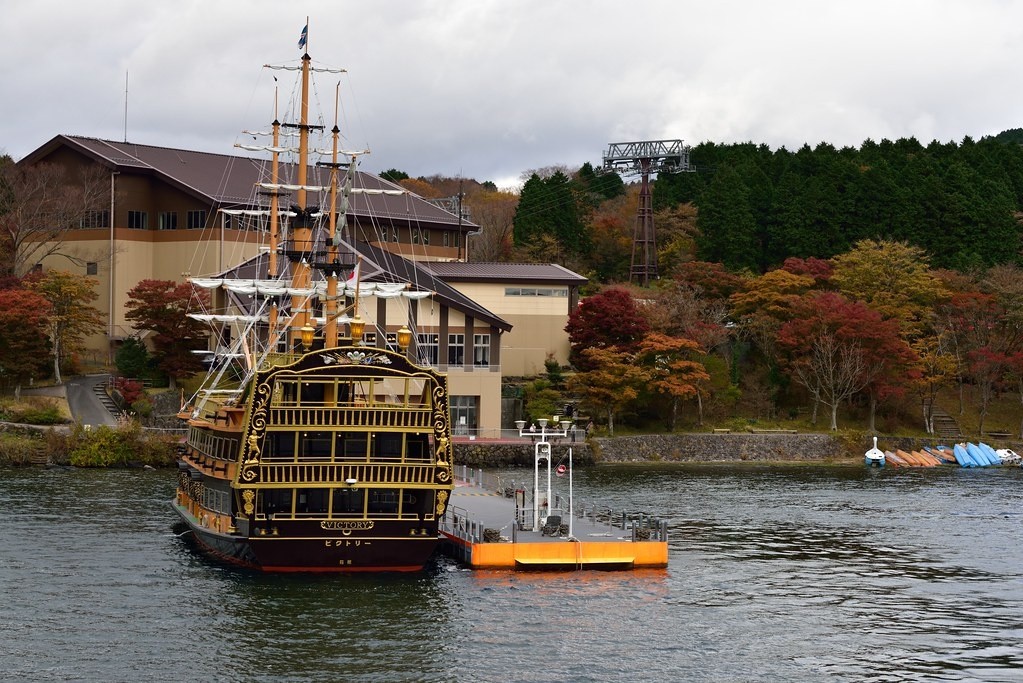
[170,15,458,583]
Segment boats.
[864,437,1022,469]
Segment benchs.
[752,429,798,434]
[712,429,731,434]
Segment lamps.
[300,323,315,352]
[397,325,412,354]
[350,314,365,347]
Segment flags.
[346,262,359,290]
